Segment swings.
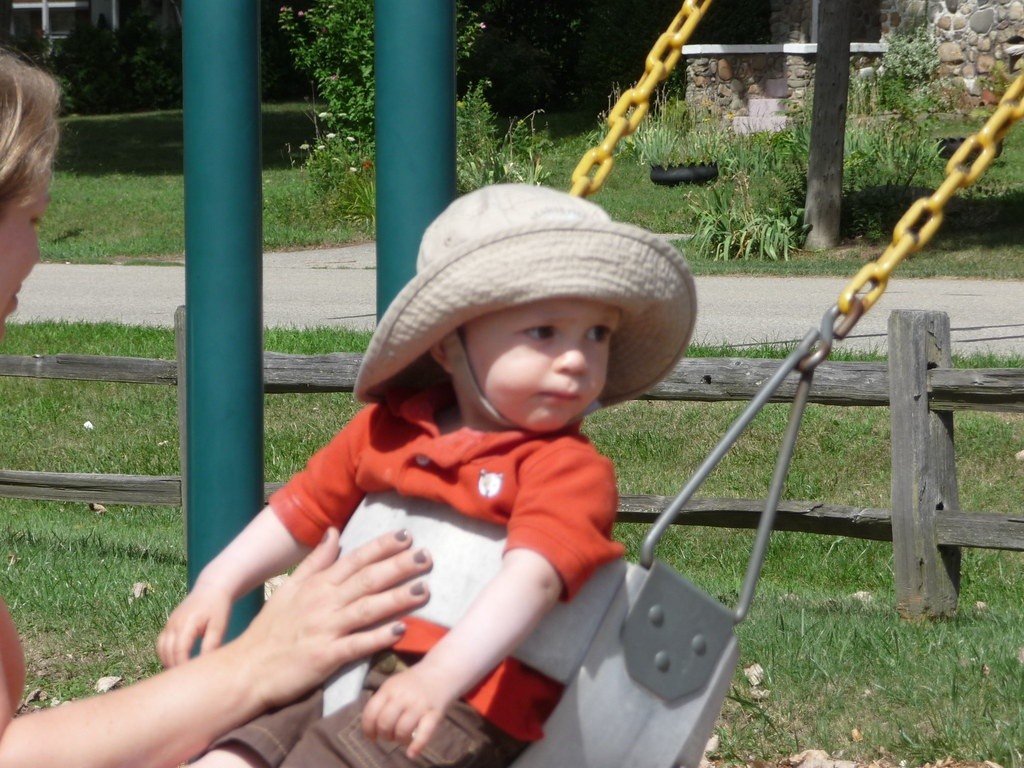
[320,1,1024,767]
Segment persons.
[0,56,435,767]
[157,184,696,767]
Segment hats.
[354,184,699,406]
